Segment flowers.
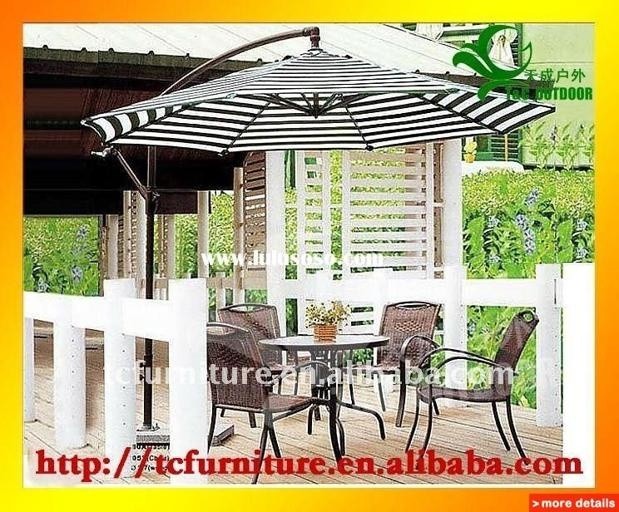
[301,303,352,330]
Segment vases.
[313,323,337,341]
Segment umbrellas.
[79,27,562,429]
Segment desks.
[252,331,390,440]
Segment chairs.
[332,299,444,427]
[206,322,345,484]
[216,301,321,437]
[406,311,536,466]
